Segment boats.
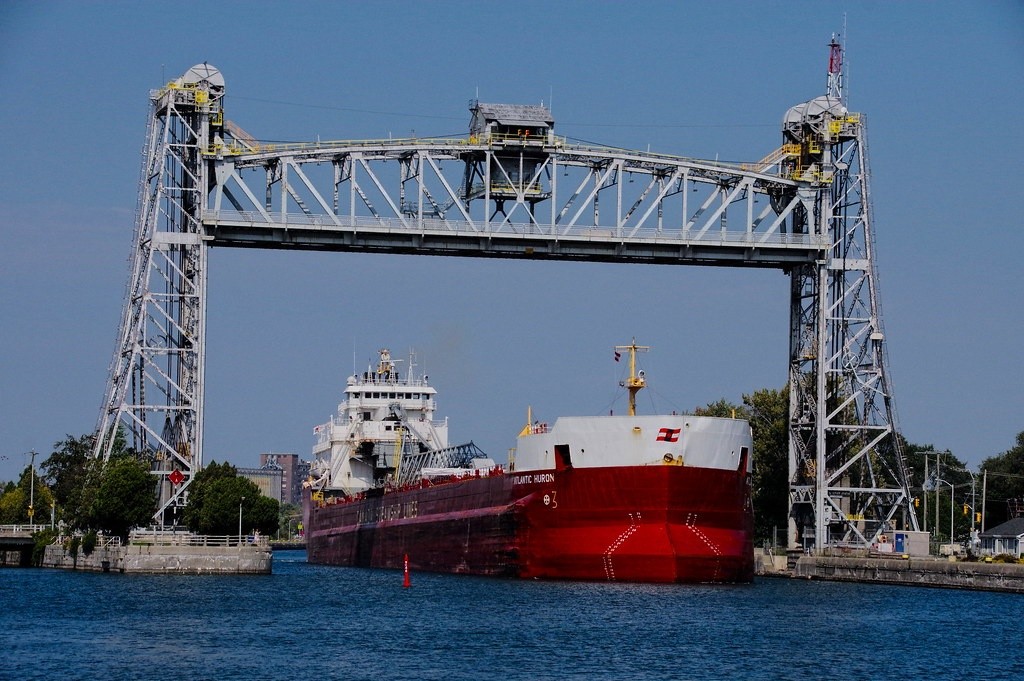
[301,337,759,588]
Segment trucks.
[940,544,965,557]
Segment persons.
[378,349,390,370]
[254,529,261,546]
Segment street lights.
[288,518,295,541]
[239,497,246,543]
[24,449,39,529]
[965,469,974,557]
[936,479,954,556]
